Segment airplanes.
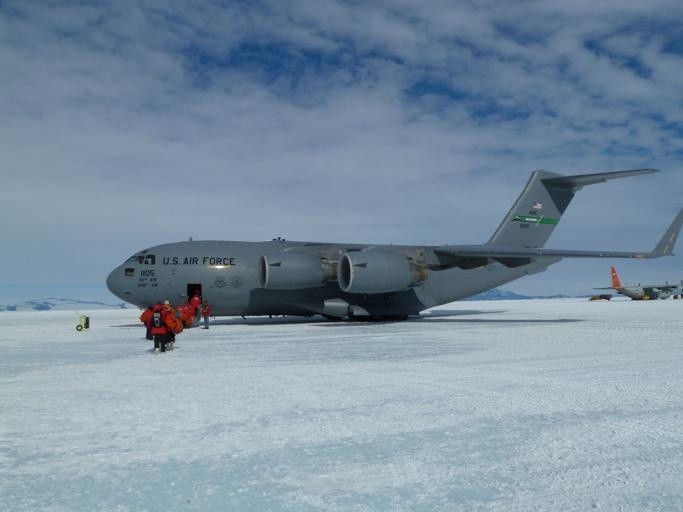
[106,168,683,328]
[592,266,683,299]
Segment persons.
[202,301,211,329]
[139,295,201,353]
